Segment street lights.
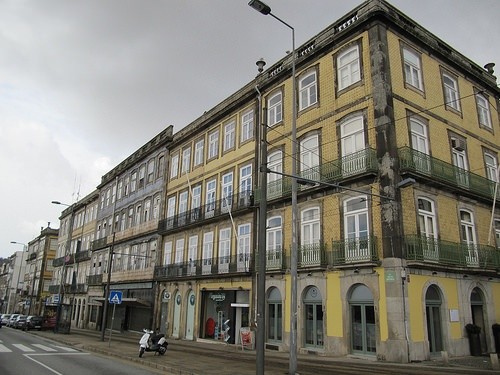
[51,201,75,332]
[10,241,26,313]
[247,0,299,375]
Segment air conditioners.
[455,139,465,150]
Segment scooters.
[138,328,168,358]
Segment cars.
[0,313,56,331]
